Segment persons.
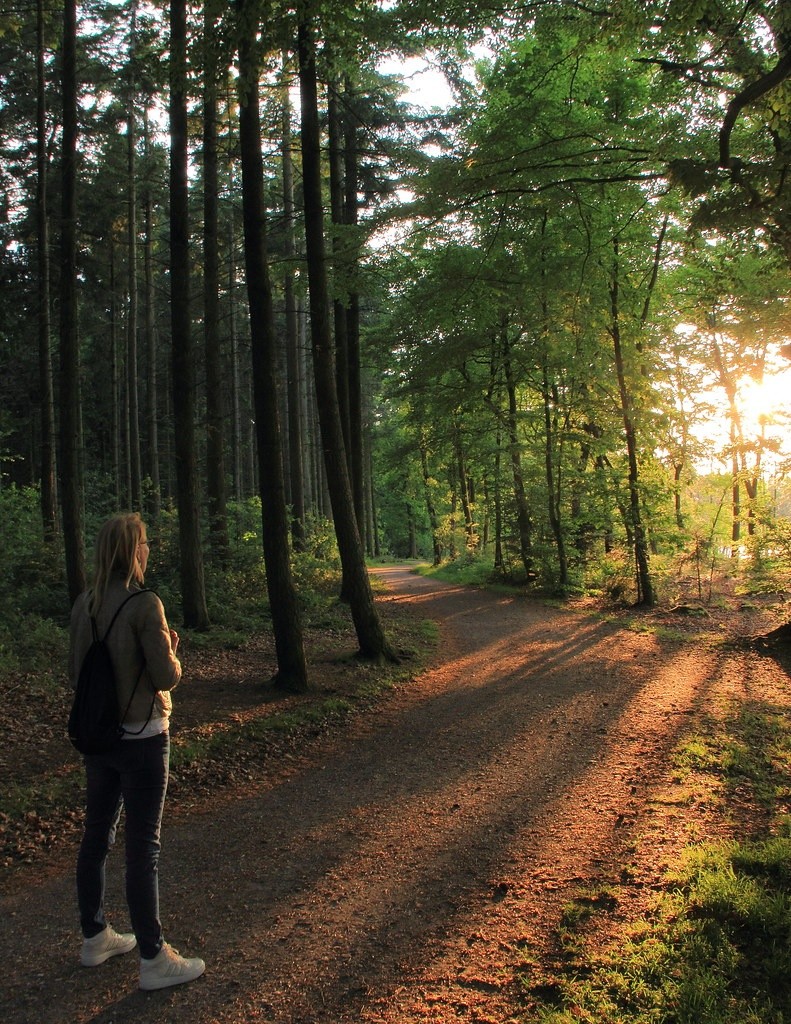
[68,512,207,990]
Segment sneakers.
[137,942,207,992]
[81,922,137,966]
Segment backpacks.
[65,590,164,759]
